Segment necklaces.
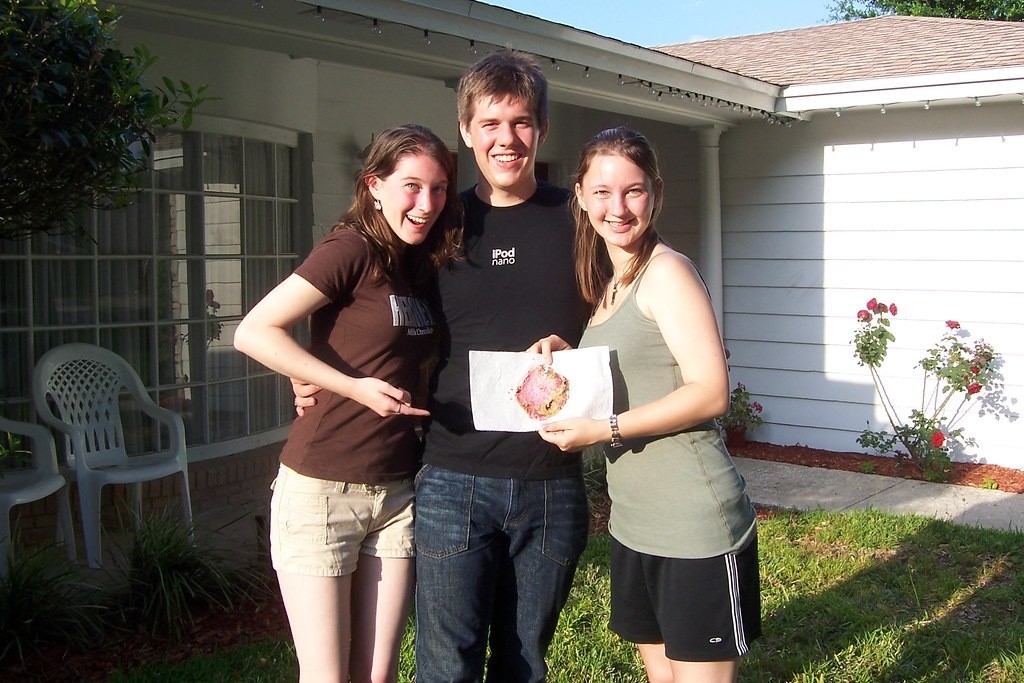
[611,265,631,305]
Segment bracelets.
[608,415,623,448]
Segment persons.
[525,126,762,683]
[288,44,731,683]
[235,124,466,683]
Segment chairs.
[30,343,196,569]
[0,417,78,580]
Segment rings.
[396,404,401,413]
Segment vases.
[726,425,747,448]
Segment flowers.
[718,381,764,434]
